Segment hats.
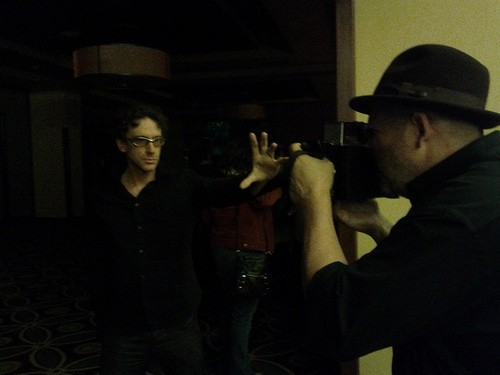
[348,44,500,128]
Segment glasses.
[124,137,167,147]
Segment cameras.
[300,122,398,201]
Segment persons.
[286,43,500,375]
[205,201,275,375]
[58,106,291,375]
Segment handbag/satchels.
[234,267,273,302]
